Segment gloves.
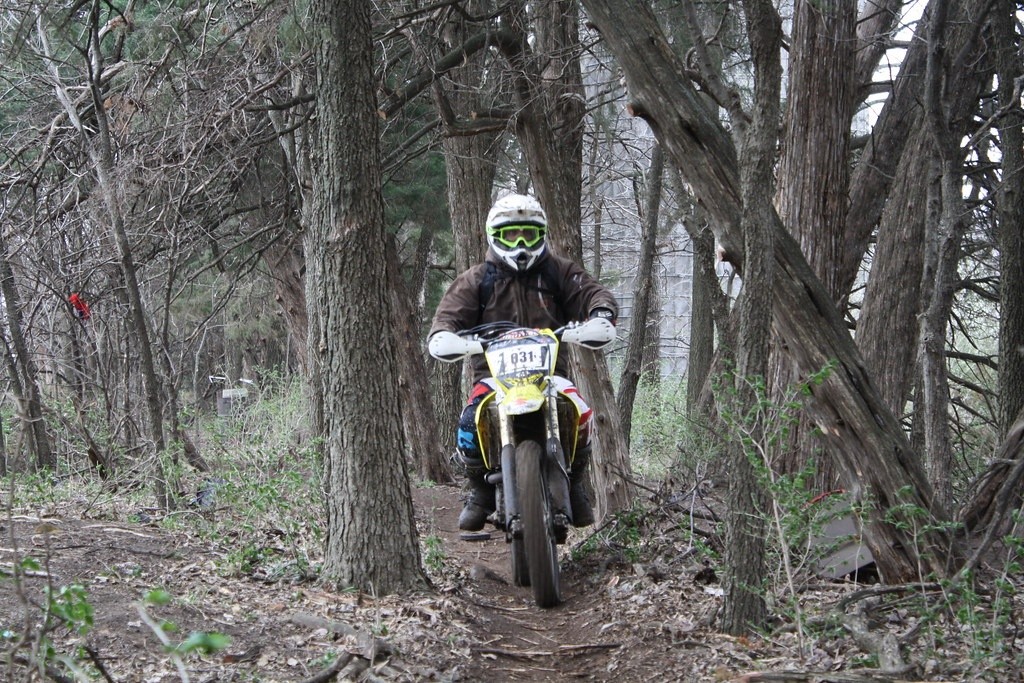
[583,309,616,326]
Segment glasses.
[486,224,547,248]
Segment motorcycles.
[428,317,617,608]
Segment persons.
[426,192,620,533]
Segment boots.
[457,446,496,532]
[569,440,595,527]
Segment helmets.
[487,193,548,271]
